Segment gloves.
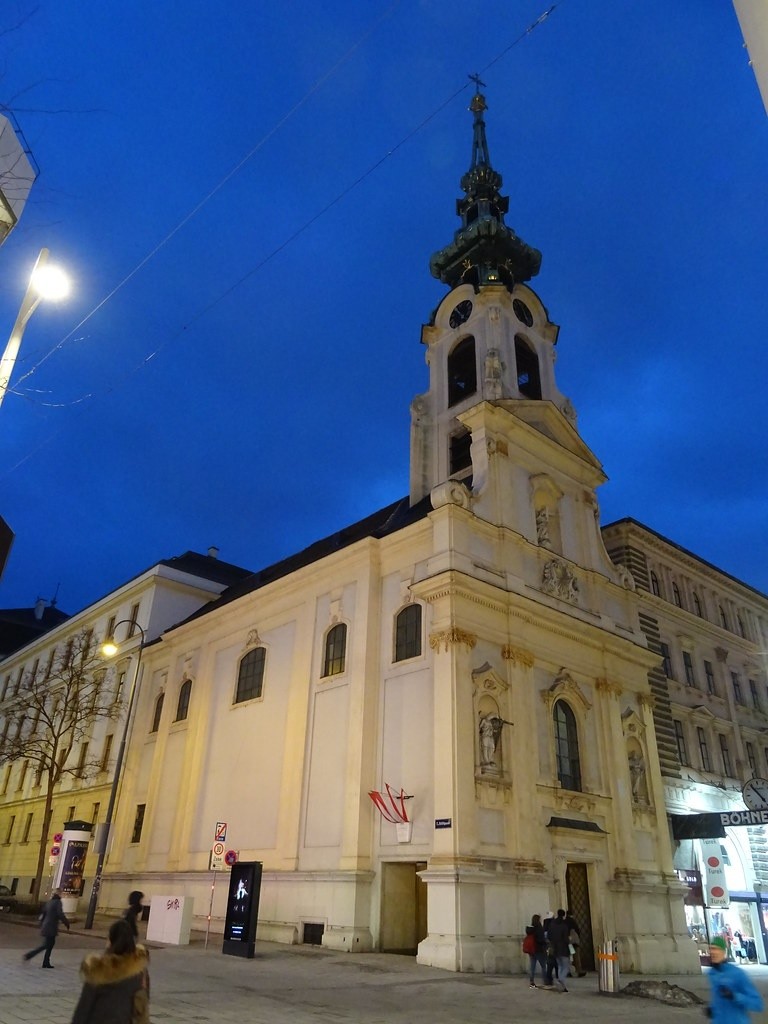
[703,1006,715,1019]
[717,985,734,1000]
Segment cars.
[0,885,18,913]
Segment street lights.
[1,246,69,405]
[85,620,144,928]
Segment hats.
[545,911,554,918]
[710,937,727,950]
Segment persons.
[23,888,70,969]
[702,936,765,1024]
[72,921,150,1024]
[526,909,587,993]
[479,711,498,766]
[628,751,644,796]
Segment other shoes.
[41,964,54,969]
[21,954,29,961]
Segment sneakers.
[529,984,540,989]
[562,988,568,992]
[543,983,557,989]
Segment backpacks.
[542,931,559,960]
[524,935,536,953]
[135,970,149,1024]
[570,929,580,947]
